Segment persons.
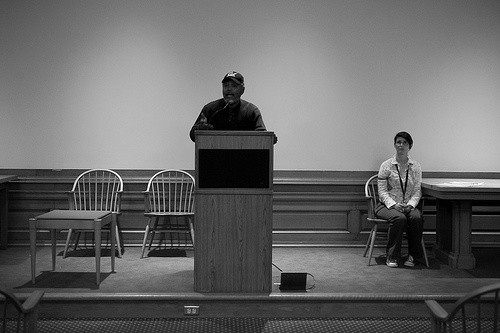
[375,132,423,267]
[189,71,277,144]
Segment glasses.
[395,141,409,146]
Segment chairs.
[425,283,500,333]
[363,175,429,268]
[140,169,196,258]
[0,289,45,333]
[62,169,124,258]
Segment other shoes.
[385,256,398,267]
[404,254,416,266]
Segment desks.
[421,178,500,269]
[29,209,115,287]
[0,174,18,250]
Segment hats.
[222,72,244,85]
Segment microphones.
[208,101,231,124]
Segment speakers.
[281,272,307,291]
